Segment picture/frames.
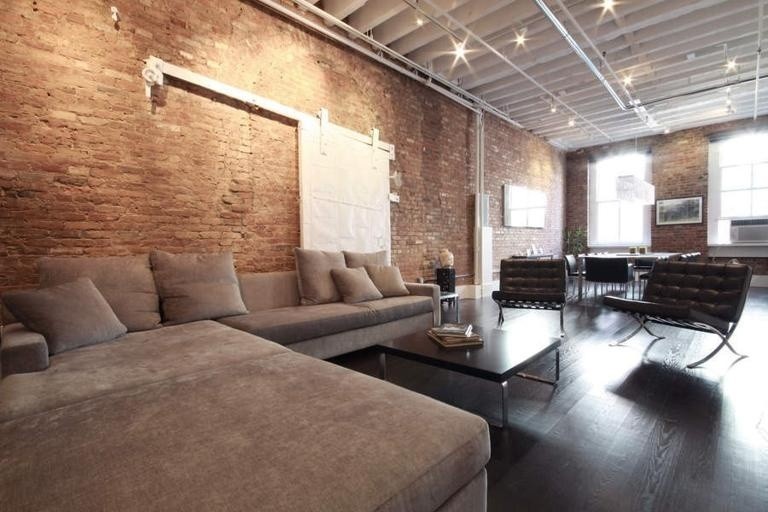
[654,194,705,227]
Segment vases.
[439,247,454,269]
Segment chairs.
[565,248,701,309]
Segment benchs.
[601,258,754,371]
[491,258,568,339]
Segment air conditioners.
[728,219,768,243]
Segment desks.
[439,290,461,325]
[511,253,553,260]
[436,268,456,306]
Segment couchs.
[0,268,494,511]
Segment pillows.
[0,275,130,358]
[342,250,389,270]
[292,247,348,306]
[36,250,163,335]
[147,245,252,327]
[363,261,411,298]
[329,266,385,305]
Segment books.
[425,322,484,350]
